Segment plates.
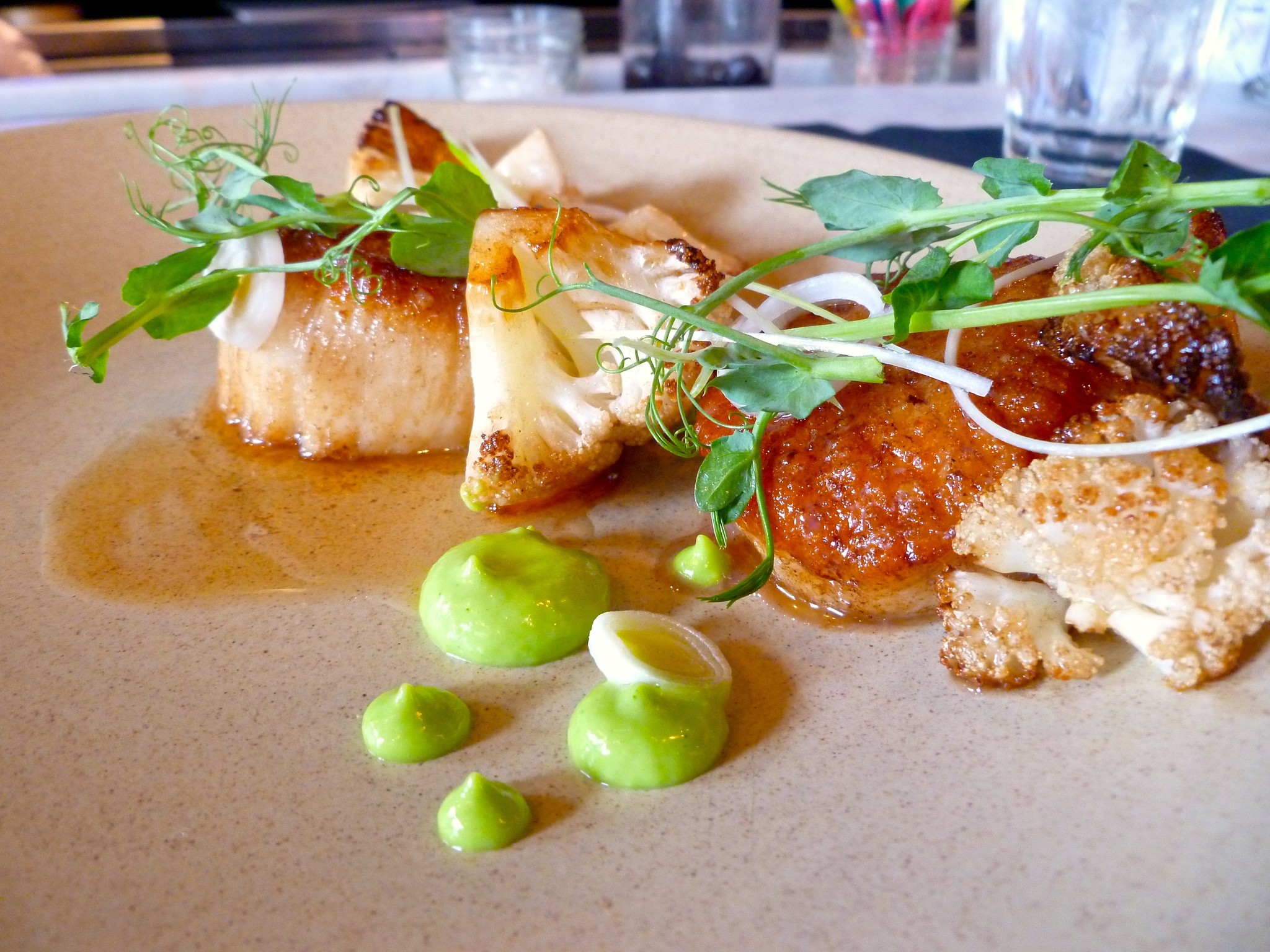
[0,101,1269,952]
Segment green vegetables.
[58,97,1270,608]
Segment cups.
[620,0,780,90]
[828,15,959,87]
[447,6,582,102]
[979,0,1228,188]
